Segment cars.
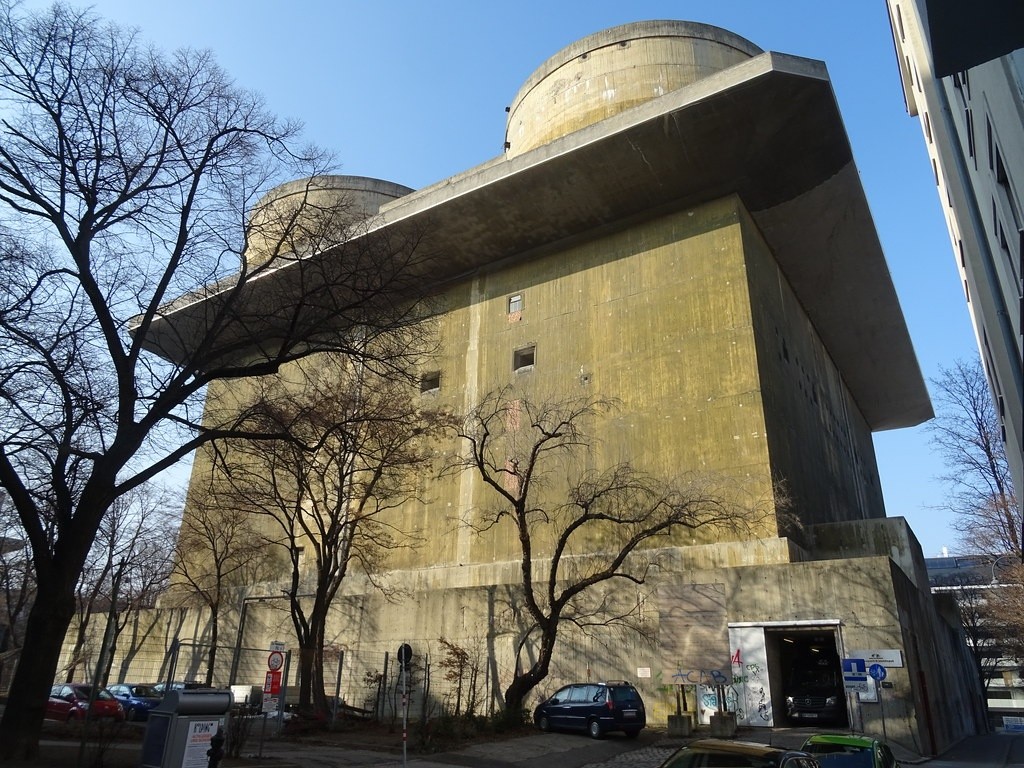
[151,681,207,696]
[100,684,166,723]
[656,736,823,767]
[44,683,125,725]
[799,734,900,768]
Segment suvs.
[534,678,647,739]
[782,661,848,728]
